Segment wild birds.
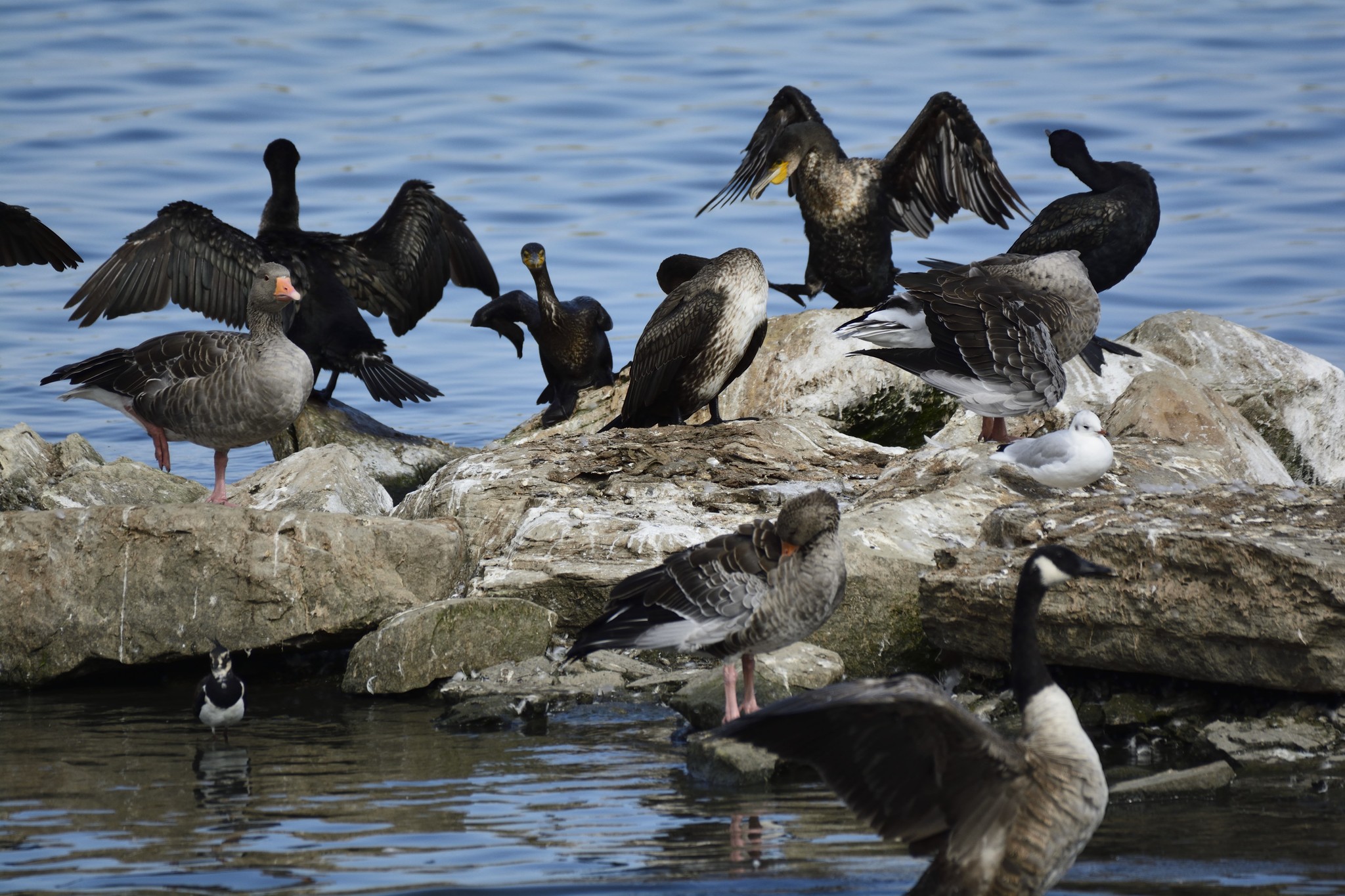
[192,643,244,744]
[592,246,769,432]
[548,486,847,725]
[693,82,1037,307]
[1,199,83,275]
[470,241,614,428]
[989,410,1114,488]
[832,128,1161,445]
[62,138,499,407]
[40,261,315,509]
[194,737,251,808]
[710,542,1120,896]
